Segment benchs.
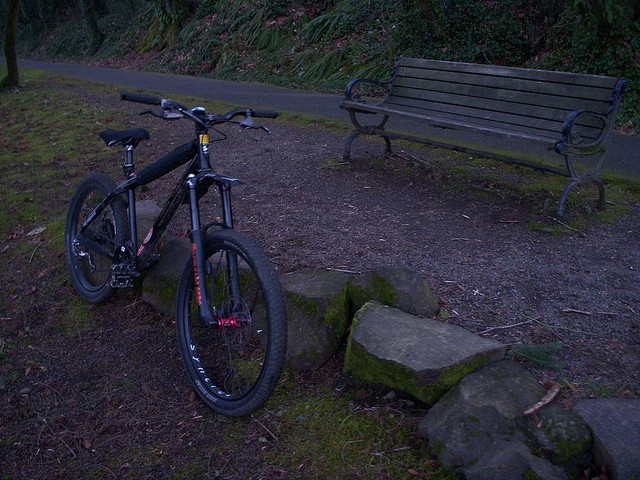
[336,56,626,234]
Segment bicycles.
[65,89,289,418]
